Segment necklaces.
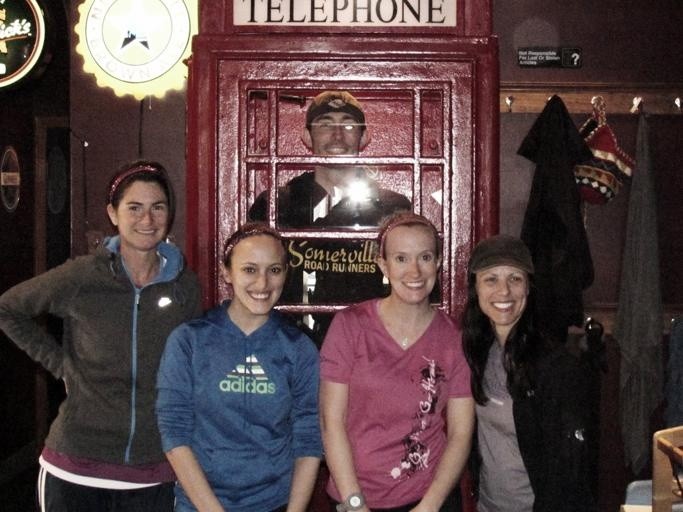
[376,304,431,347]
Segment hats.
[470,234,535,275]
[306,90,365,127]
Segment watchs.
[334,491,365,511]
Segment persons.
[151,219,324,510]
[458,233,597,512]
[1,160,201,511]
[247,87,440,349]
[318,208,479,512]
